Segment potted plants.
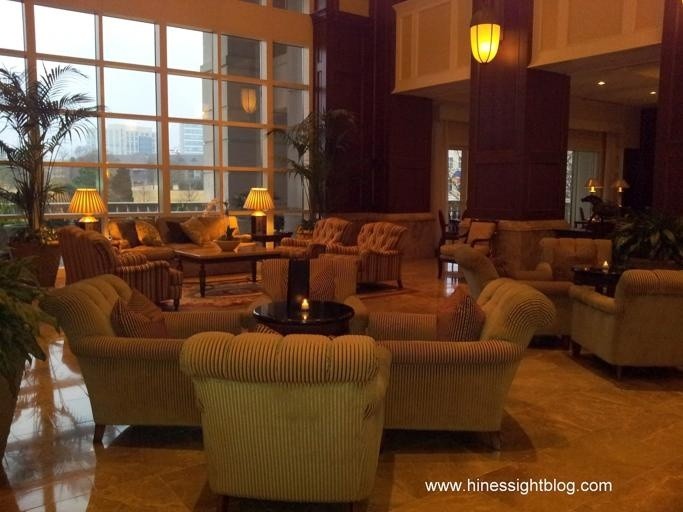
[613,201,682,275]
[0,64,113,293]
[0,242,62,469]
[212,197,241,251]
[261,107,359,239]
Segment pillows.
[433,283,486,341]
[255,322,283,335]
[281,260,336,302]
[131,217,165,246]
[178,215,218,245]
[111,289,169,340]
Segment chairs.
[322,223,407,291]
[432,217,500,281]
[275,217,355,264]
[433,217,473,257]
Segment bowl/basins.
[213,239,240,252]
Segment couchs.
[37,272,246,442]
[364,277,556,452]
[246,253,371,336]
[177,333,393,504]
[533,237,613,279]
[101,211,257,279]
[452,247,595,349]
[56,224,185,312]
[568,268,683,381]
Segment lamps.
[237,88,260,115]
[583,177,603,201]
[64,187,109,235]
[470,23,501,65]
[242,186,276,240]
[611,180,631,208]
